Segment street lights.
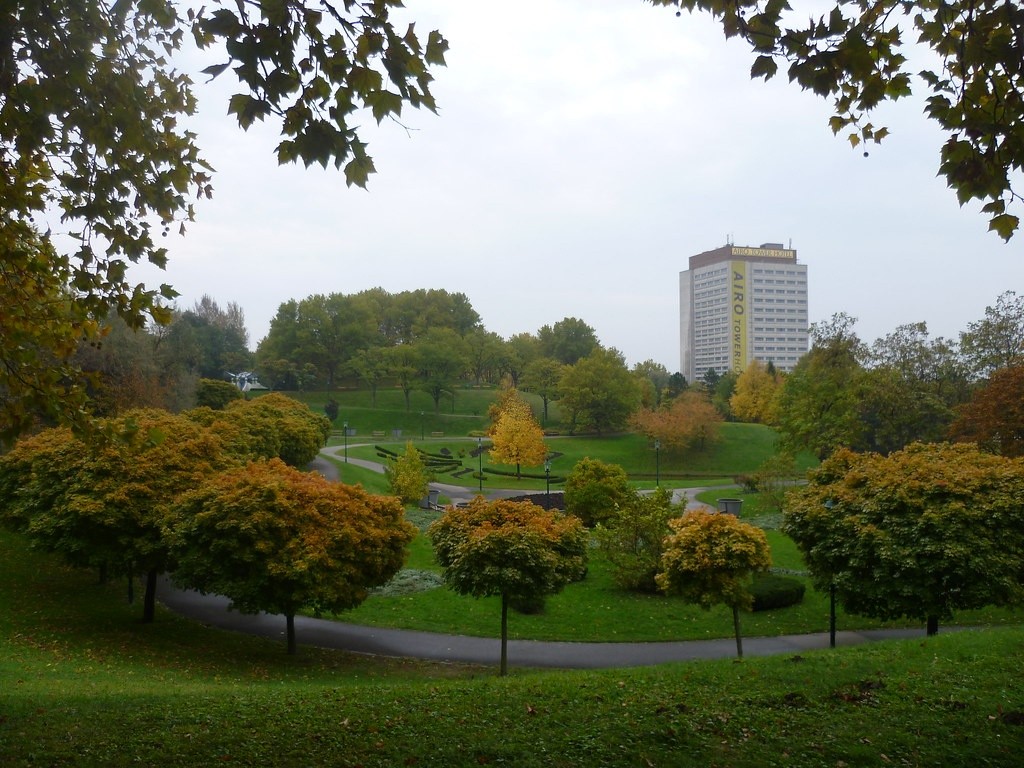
[419,409,424,441]
[478,438,483,491]
[655,440,661,490]
[344,420,348,463]
[544,457,552,510]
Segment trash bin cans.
[716,498,743,518]
[419,489,440,508]
